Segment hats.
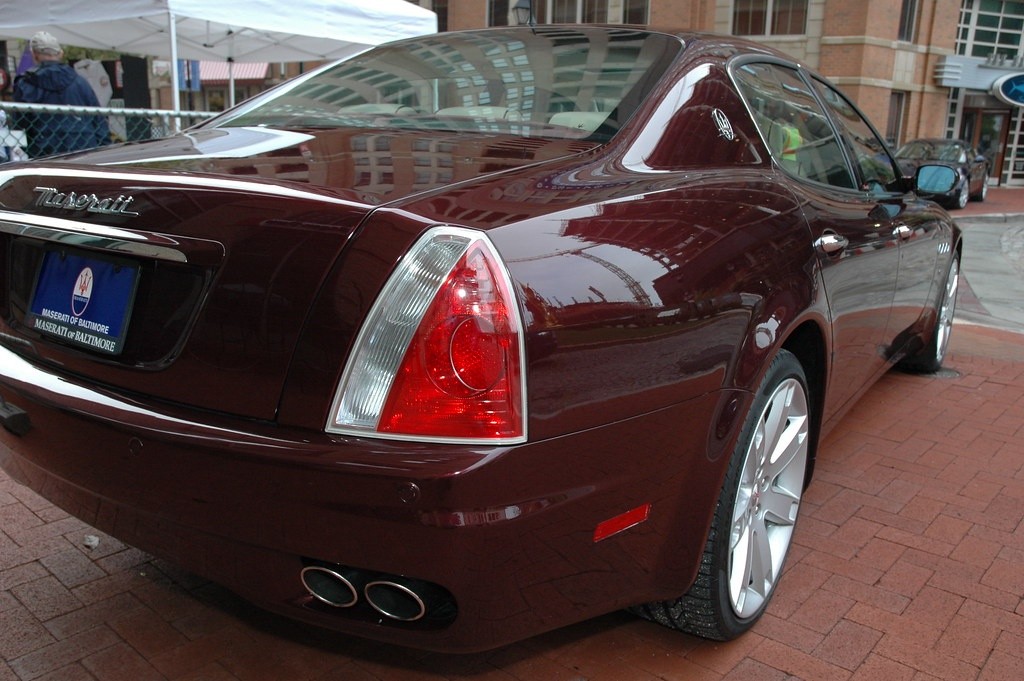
[29,31,59,55]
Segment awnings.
[183,61,269,86]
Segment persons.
[12,30,112,159]
[776,112,803,169]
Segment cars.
[1,22,960,656]
[891,136,990,210]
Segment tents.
[0,0,439,133]
